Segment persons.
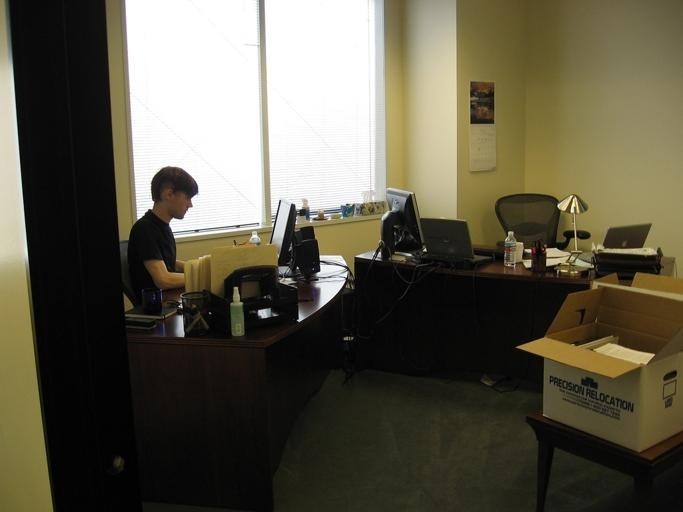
[128,165,200,307]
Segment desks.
[352,247,677,392]
[122,254,347,511]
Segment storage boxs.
[515,270,682,452]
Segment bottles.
[502,231,517,267]
[247,231,262,245]
[531,239,548,271]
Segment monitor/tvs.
[380,187,426,261]
[269,198,316,278]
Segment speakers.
[295,237,321,283]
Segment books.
[570,333,656,366]
[124,306,178,319]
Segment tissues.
[355,191,386,216]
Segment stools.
[524,403,683,512]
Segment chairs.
[117,241,139,309]
[494,194,589,251]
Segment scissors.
[535,239,546,254]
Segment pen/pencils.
[532,248,536,258]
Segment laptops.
[577,223,652,264]
[420,217,493,268]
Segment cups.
[512,241,524,263]
[340,201,386,217]
[141,286,163,316]
